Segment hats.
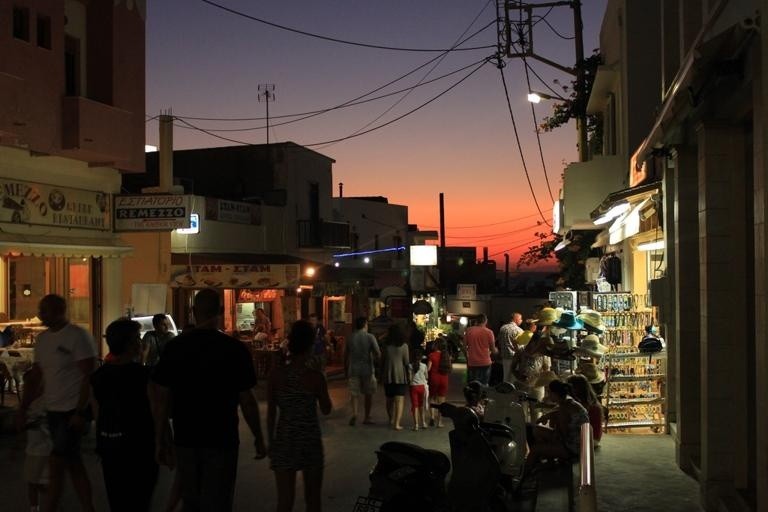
[544,338,576,361]
[531,306,565,326]
[534,370,559,389]
[575,363,605,384]
[552,310,584,330]
[573,334,610,357]
[566,374,588,388]
[576,308,606,331]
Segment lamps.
[525,89,578,112]
[588,174,665,230]
[636,237,666,254]
[552,222,573,254]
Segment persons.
[280,312,331,379]
[465,306,607,426]
[266,318,332,511]
[24,369,50,512]
[345,317,453,431]
[157,287,266,512]
[142,314,176,370]
[14,293,99,512]
[251,308,272,335]
[461,373,604,473]
[89,319,159,512]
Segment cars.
[127,312,181,343]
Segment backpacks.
[599,252,622,293]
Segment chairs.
[222,322,348,387]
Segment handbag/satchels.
[637,329,664,353]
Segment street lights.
[525,88,588,165]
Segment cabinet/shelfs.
[590,291,670,435]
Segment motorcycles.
[346,377,559,511]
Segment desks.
[0,320,49,405]
